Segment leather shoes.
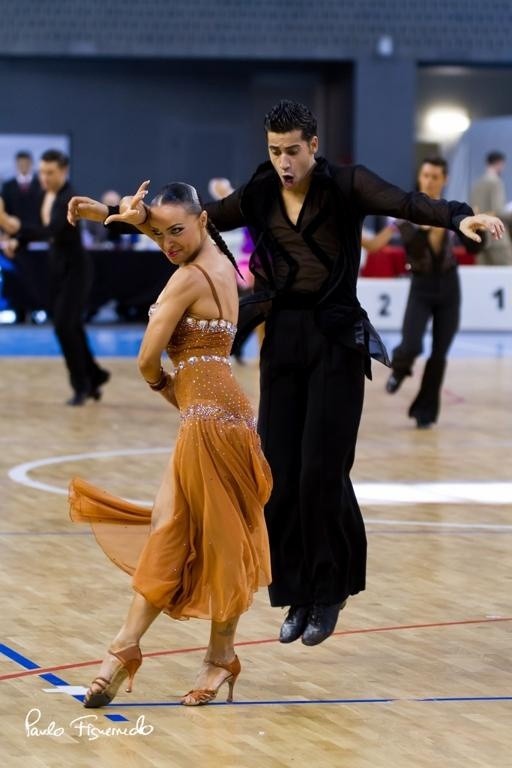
[69,387,100,407]
[301,600,348,646]
[279,598,309,641]
[388,371,406,393]
[416,420,432,430]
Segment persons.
[67,101,506,647]
[82,179,272,709]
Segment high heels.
[181,655,240,706]
[83,645,142,708]
